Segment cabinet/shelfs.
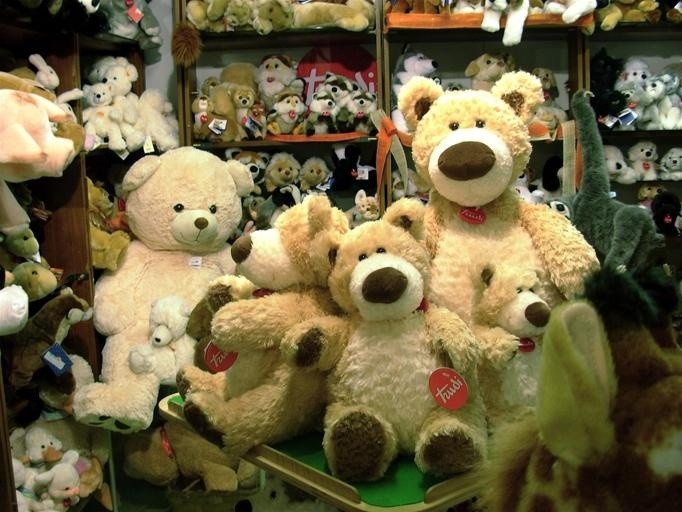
[381,0,584,238]
[170,0,381,233]
[586,0,682,255]
[77,32,149,372]
[0,0,119,512]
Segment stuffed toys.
[0,0,682,512]
[1,0,682,512]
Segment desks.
[154,368,493,511]
